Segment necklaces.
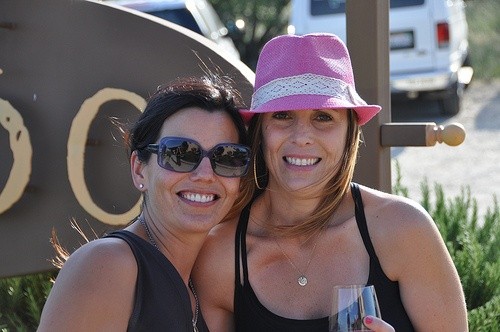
[268,204,322,287]
[135,218,203,332]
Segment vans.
[291,0,473,117]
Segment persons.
[187,33,470,332]
[35,50,256,332]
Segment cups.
[328,284,381,332]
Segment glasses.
[142,136,254,179]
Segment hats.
[238,33,382,126]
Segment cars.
[111,0,247,59]
